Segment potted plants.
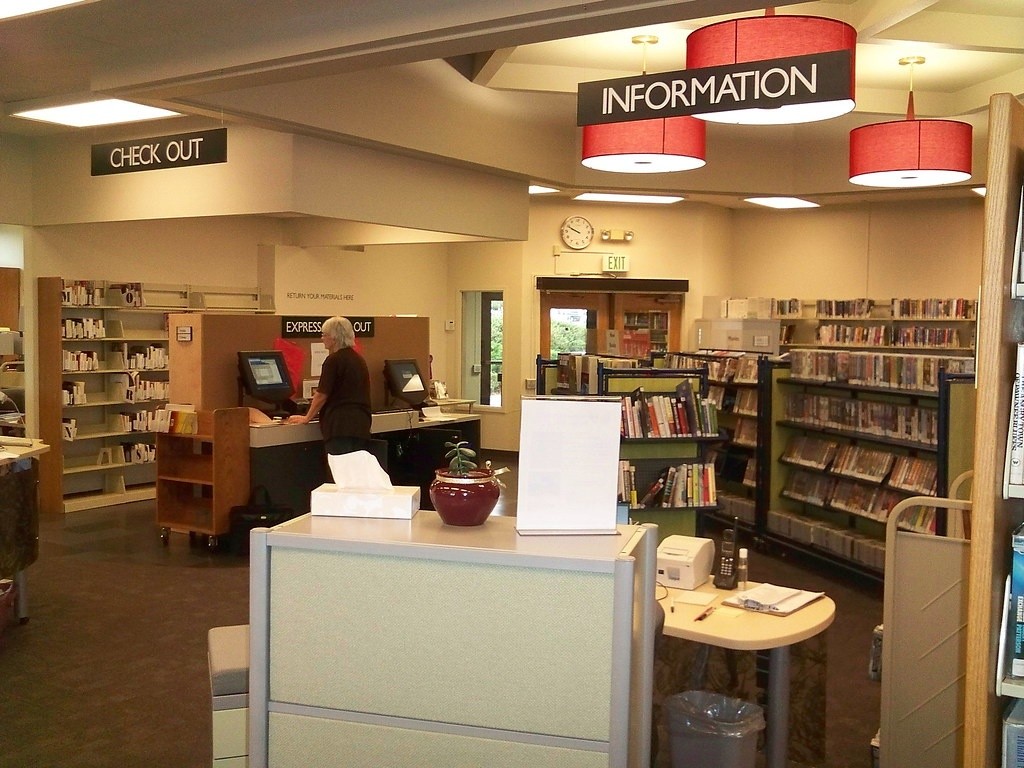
[429,441,500,526]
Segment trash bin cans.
[666,691,764,768]
[0,578,15,633]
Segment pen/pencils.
[671,598,674,613]
[0,441,33,447]
[694,607,716,622]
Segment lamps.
[600,228,634,242]
[686,7,857,126]
[581,35,707,174]
[849,57,973,188]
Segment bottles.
[737,548,749,590]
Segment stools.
[207,625,249,767]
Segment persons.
[287,316,372,484]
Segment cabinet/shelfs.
[536,298,977,584]
[155,403,250,553]
[39,276,276,514]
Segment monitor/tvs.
[237,350,295,404]
[383,359,428,405]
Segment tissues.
[310,449,422,521]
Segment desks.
[0,437,50,623]
[249,399,482,468]
[655,575,835,768]
[247,511,659,768]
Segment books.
[871,727,880,768]
[1009,522,1024,678]
[1010,341,1024,486]
[59,276,106,442]
[556,296,980,570]
[111,282,144,307]
[121,345,198,464]
[1001,697,1024,768]
[430,379,448,399]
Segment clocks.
[561,215,594,250]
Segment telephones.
[713,516,738,590]
